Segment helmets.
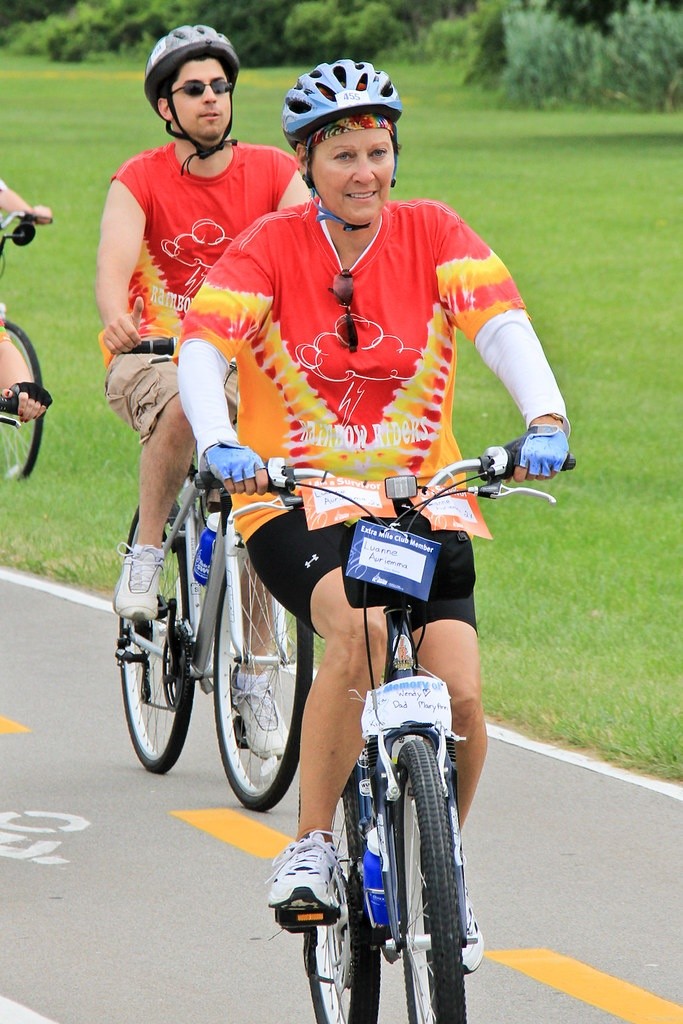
[280,58,403,151]
[144,24,240,122]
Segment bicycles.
[108,339,322,813]
[186,433,579,1024]
[1,209,55,478]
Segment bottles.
[362,827,389,928]
[192,512,219,586]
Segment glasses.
[168,78,233,96]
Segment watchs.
[550,414,564,424]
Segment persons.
[97,25,311,762]
[0,315,52,422]
[175,58,567,970]
[0,179,51,225]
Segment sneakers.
[233,663,289,761]
[265,830,347,911]
[113,542,165,620]
[463,885,483,975]
